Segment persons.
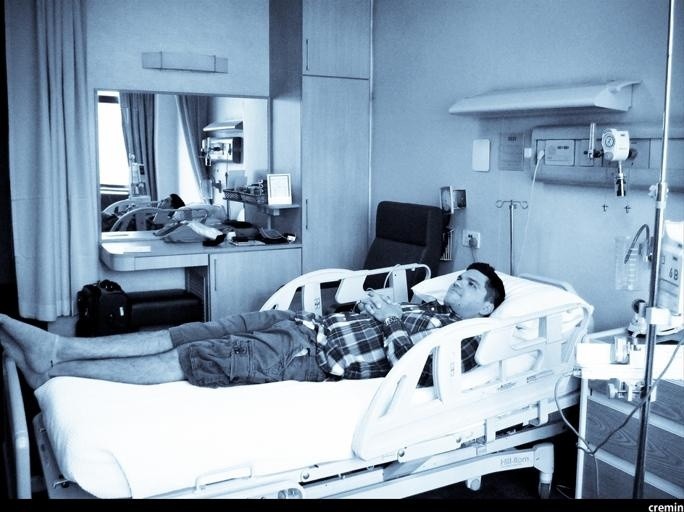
[102,192,185,232]
[0,263,505,392]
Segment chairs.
[269,201,444,315]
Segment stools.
[126,289,203,333]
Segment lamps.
[143,52,228,73]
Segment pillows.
[410,267,582,365]
[183,200,212,217]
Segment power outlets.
[462,230,480,249]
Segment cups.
[609,333,631,365]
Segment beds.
[101,200,228,232]
[4,263,593,500]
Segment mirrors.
[94,88,273,244]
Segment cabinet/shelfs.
[269,0,373,79]
[208,247,302,323]
[271,77,371,271]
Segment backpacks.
[74,281,133,337]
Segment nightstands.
[574,327,684,500]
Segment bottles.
[200,179,212,200]
[615,234,640,292]
[587,122,596,158]
[614,173,626,197]
